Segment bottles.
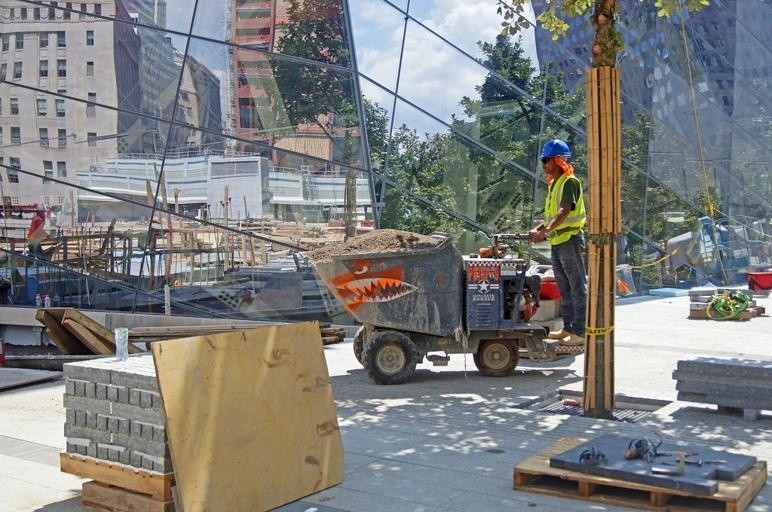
[35,293,42,306]
[44,295,51,307]
[53,294,59,307]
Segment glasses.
[541,158,548,162]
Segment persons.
[27,206,50,253]
[528,139,586,346]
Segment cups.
[114,327,130,361]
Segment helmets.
[537,139,571,158]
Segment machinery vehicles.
[299,230,584,386]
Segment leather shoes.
[548,330,587,345]
